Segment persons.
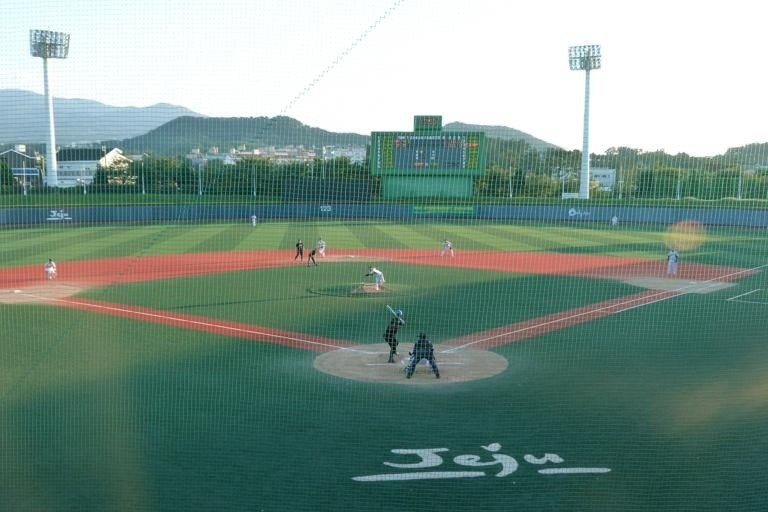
[366,266,386,290]
[251,214,256,227]
[318,236,327,258]
[293,239,304,263]
[612,215,618,227]
[43,258,57,281]
[382,310,405,363]
[405,332,439,378]
[439,238,454,257]
[307,249,317,267]
[666,248,679,279]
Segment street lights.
[567,43,602,199]
[28,28,70,187]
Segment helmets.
[396,310,402,316]
[419,333,427,339]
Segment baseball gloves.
[365,273,369,276]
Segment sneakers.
[436,374,440,378]
[407,374,411,379]
[388,360,395,363]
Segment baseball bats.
[386,305,402,321]
